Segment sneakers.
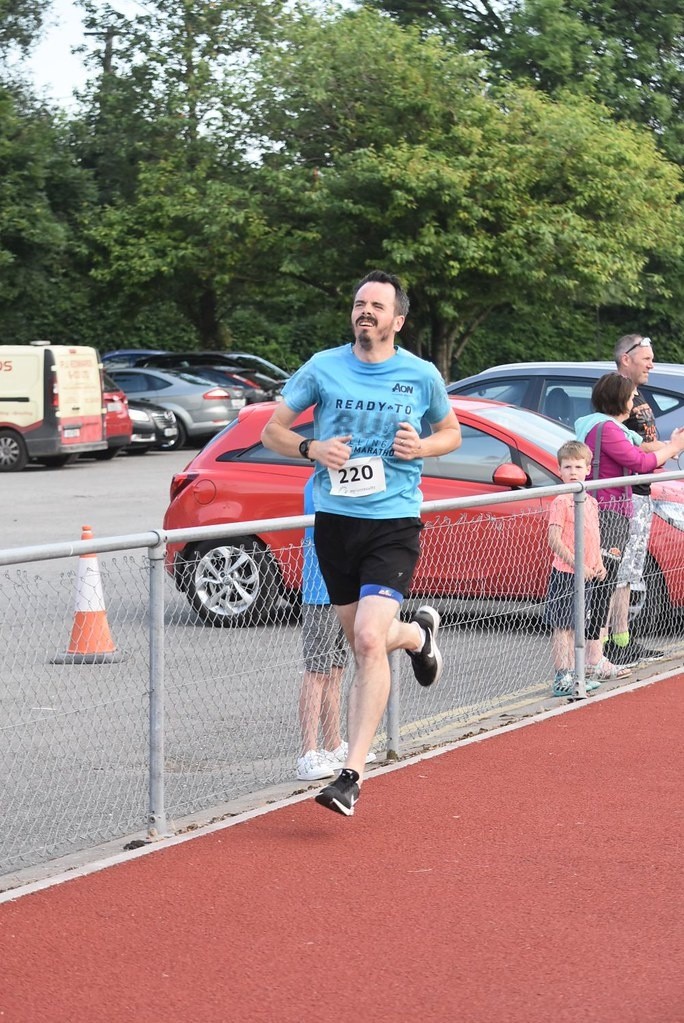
[321,740,376,770]
[553,675,592,696]
[604,640,638,666]
[315,768,360,816]
[627,637,664,661]
[297,752,334,781]
[405,606,442,686]
[585,679,601,688]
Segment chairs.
[544,388,571,423]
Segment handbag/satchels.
[597,510,629,566]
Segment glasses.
[626,338,650,354]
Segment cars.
[155,391,684,642]
[441,359,684,477]
[66,372,132,463]
[101,346,292,455]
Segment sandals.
[585,656,632,681]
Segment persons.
[584,372,684,679]
[260,269,461,815]
[547,440,605,695]
[297,471,377,780]
[603,333,679,668]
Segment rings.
[401,438,406,445]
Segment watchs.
[298,437,317,462]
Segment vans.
[0,340,110,474]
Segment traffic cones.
[44,525,129,666]
